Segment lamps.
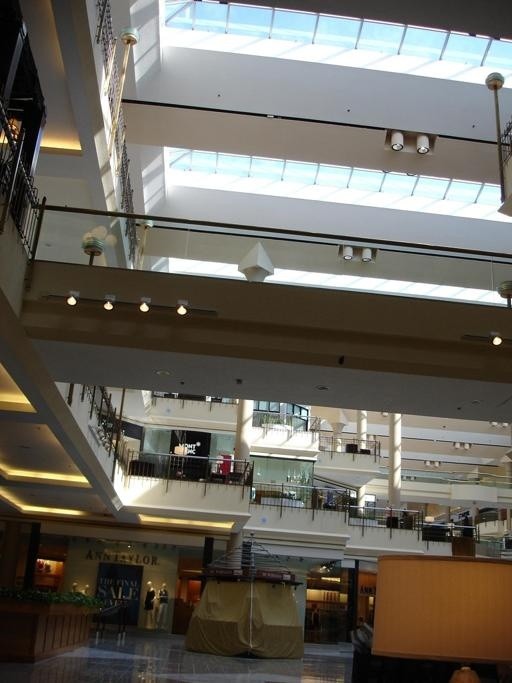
[369,551,512,683]
[455,441,471,450]
[390,129,432,156]
[491,420,510,430]
[341,245,375,264]
[426,459,440,467]
[67,289,191,320]
[488,328,504,348]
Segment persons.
[143,581,156,630]
[158,582,170,630]
[70,582,78,592]
[81,584,90,595]
[312,603,323,643]
